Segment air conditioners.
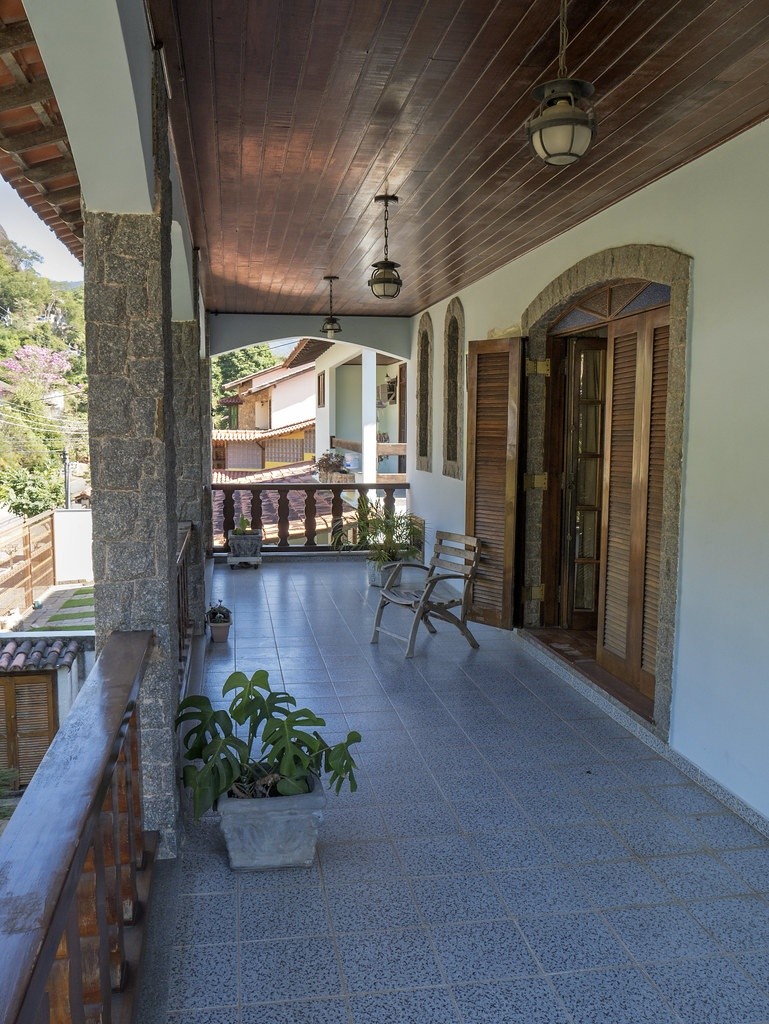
[376,384,388,403]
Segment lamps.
[367,195,405,300]
[524,0,598,169]
[385,373,397,387]
[319,276,343,339]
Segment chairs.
[369,530,482,658]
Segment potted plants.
[175,670,361,872]
[228,513,264,556]
[329,492,434,589]
[313,448,355,491]
[206,599,233,643]
